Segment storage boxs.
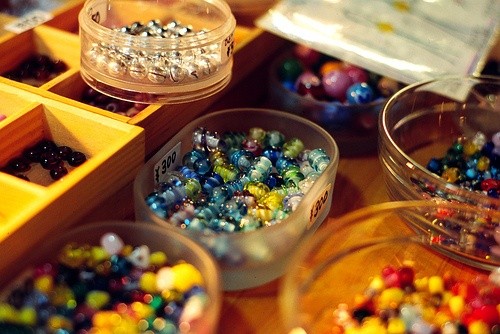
[0,221,224,334]
[377,74,500,271]
[279,199,500,334]
[79,1,237,105]
[135,108,339,292]
